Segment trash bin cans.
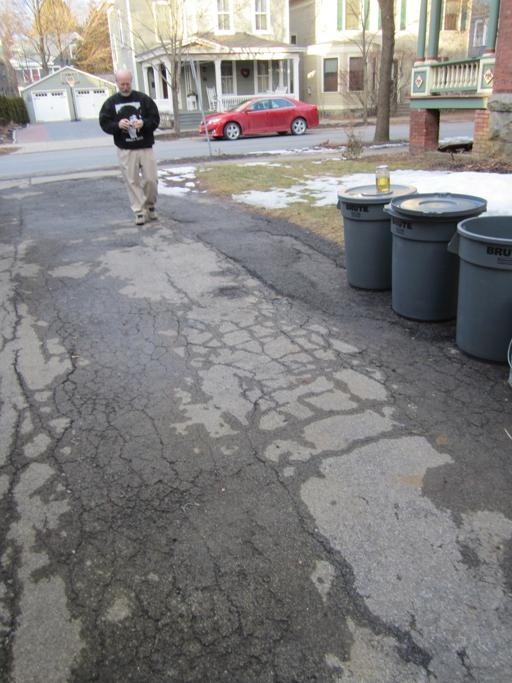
[186,93,197,111]
[338,184,512,367]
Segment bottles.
[375,164,391,193]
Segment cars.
[200,94,321,139]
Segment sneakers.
[134,211,146,225]
[144,200,158,220]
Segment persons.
[99,68,160,225]
